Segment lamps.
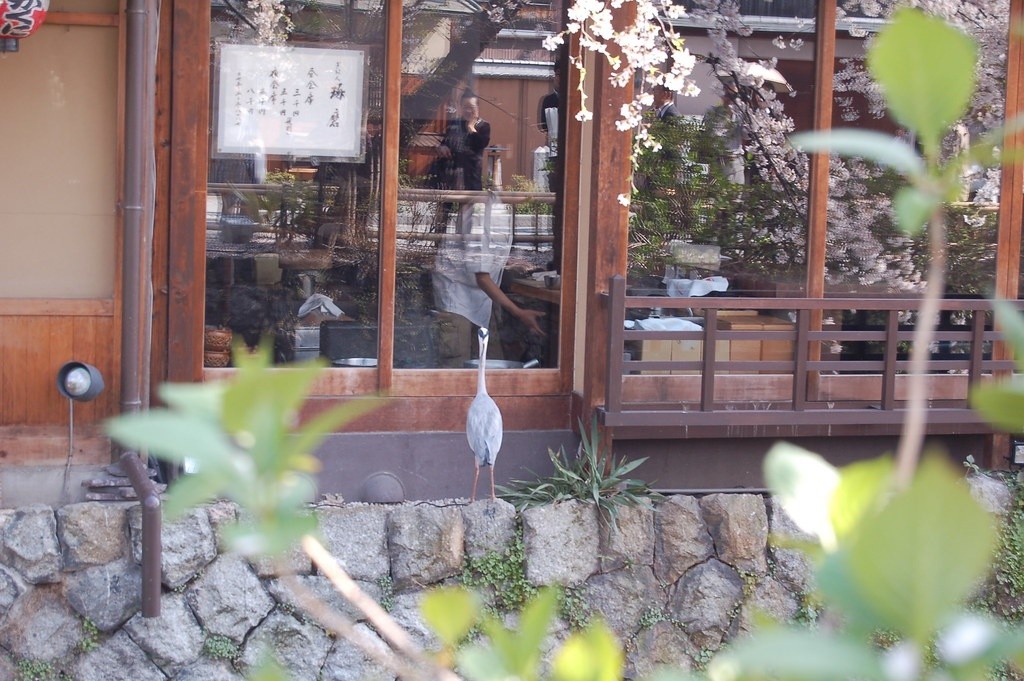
[54,358,105,493]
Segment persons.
[644,87,684,195]
[435,94,491,247]
[744,82,783,185]
[429,196,548,368]
[536,57,563,270]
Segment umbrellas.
[715,61,793,95]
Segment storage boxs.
[633,315,795,374]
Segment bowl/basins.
[544,275,561,289]
[331,358,395,367]
[288,167,318,181]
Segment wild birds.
[466,327,503,505]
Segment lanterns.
[0,0,49,52]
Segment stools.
[279,248,338,300]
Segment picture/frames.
[210,36,370,164]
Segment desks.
[494,265,710,370]
